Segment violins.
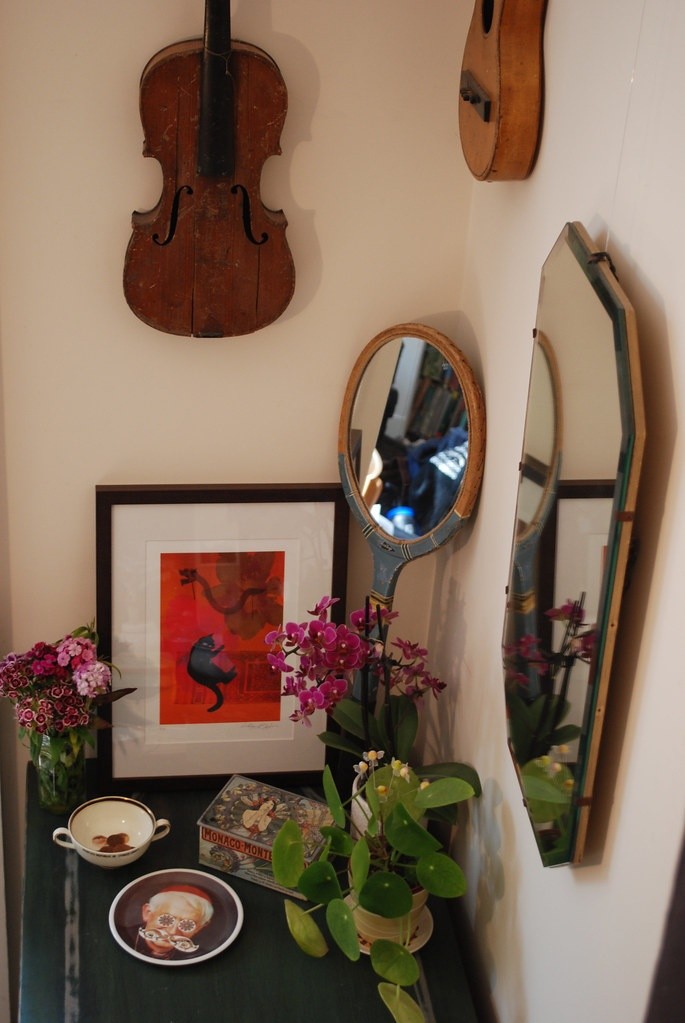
[117,0,297,342]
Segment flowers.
[499,592,602,826]
[266,594,475,1023]
[0,620,138,807]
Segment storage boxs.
[198,775,338,902]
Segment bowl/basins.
[53,796,171,869]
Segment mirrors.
[336,323,487,714]
[501,223,647,869]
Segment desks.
[16,758,480,1023]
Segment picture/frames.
[96,483,348,786]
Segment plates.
[108,868,244,965]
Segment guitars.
[455,0,548,184]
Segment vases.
[32,731,86,812]
[349,771,429,841]
[347,870,429,945]
